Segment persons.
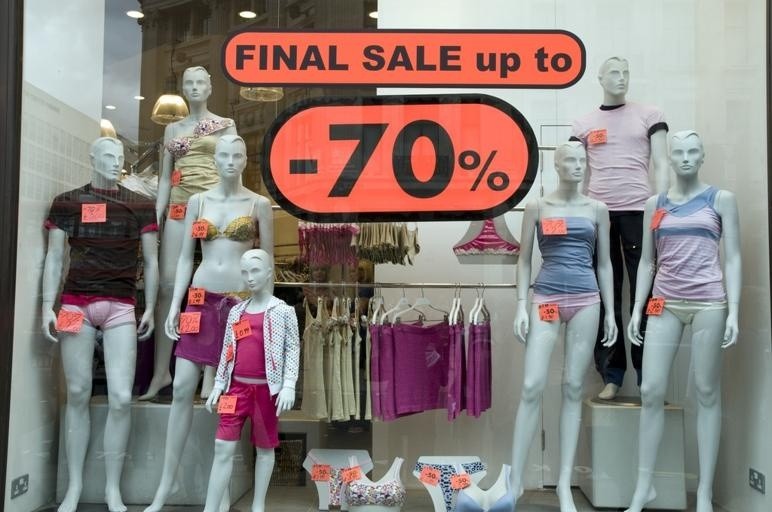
[139,66,236,401]
[150,135,277,488]
[39,137,158,512]
[199,248,299,512]
[626,130,742,512]
[574,55,668,400]
[508,140,620,512]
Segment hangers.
[272,262,307,283]
[304,281,490,328]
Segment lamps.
[150,46,190,125]
